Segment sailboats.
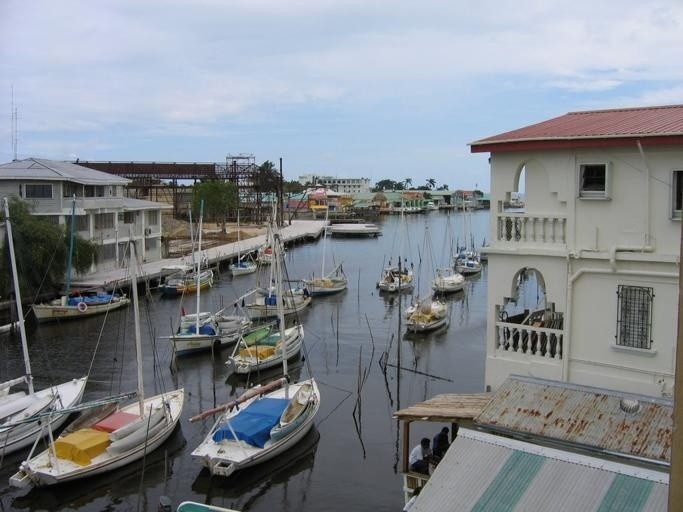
[153,192,348,374]
[1,196,88,458]
[378,190,491,334]
[30,199,131,320]
[9,227,185,499]
[188,194,322,478]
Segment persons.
[432,426,450,456]
[409,438,440,474]
